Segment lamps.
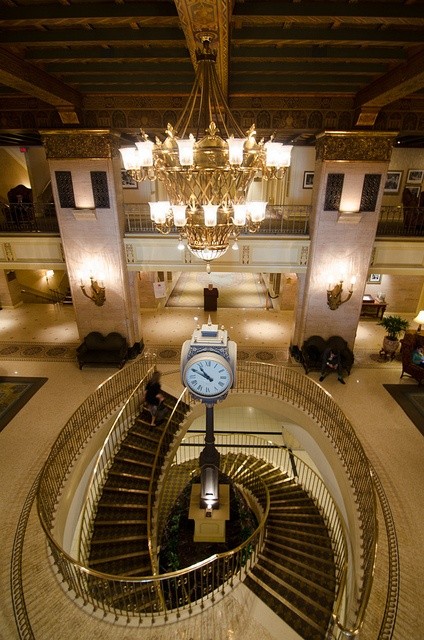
[118,27,292,274]
[79,277,106,306]
[412,311,424,331]
[326,275,356,310]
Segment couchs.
[400,332,424,387]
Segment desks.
[359,297,386,321]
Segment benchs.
[76,332,129,370]
[301,335,354,376]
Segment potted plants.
[377,313,409,361]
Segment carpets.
[383,384,424,437]
[0,376,48,432]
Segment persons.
[12,192,31,221]
[412,344,424,368]
[145,371,165,426]
[319,348,345,384]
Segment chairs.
[7,185,34,221]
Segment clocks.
[180,314,237,408]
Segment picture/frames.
[303,171,314,189]
[404,185,421,208]
[382,170,403,194]
[121,168,138,189]
[367,273,382,284]
[405,168,424,185]
